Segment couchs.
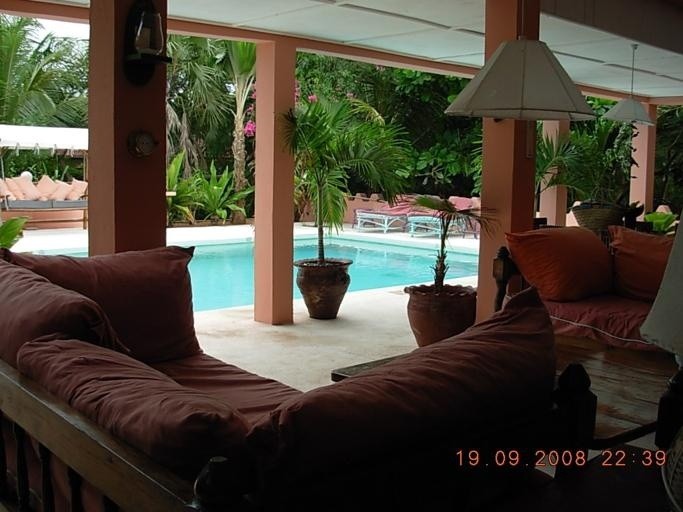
[489,227,679,355]
[0,243,597,512]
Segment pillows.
[0,173,89,202]
[501,224,675,309]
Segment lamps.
[443,2,657,129]
[637,210,683,450]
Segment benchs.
[1,178,88,232]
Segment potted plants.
[401,190,499,349]
[287,102,411,321]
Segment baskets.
[571,188,624,229]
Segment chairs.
[352,207,481,240]
